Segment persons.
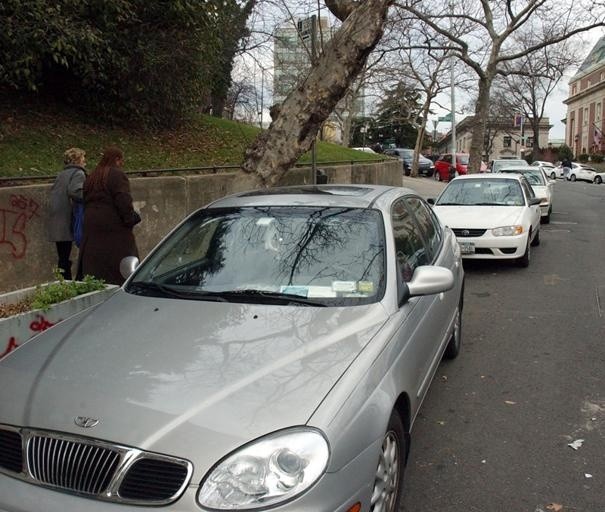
[45,148,89,282]
[75,148,140,289]
[561,156,572,182]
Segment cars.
[496,166,557,224]
[426,172,543,268]
[1,183,466,511]
[349,147,605,185]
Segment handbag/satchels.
[119,210,142,228]
[68,169,83,248]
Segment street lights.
[512,95,531,160]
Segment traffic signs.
[438,116,452,121]
